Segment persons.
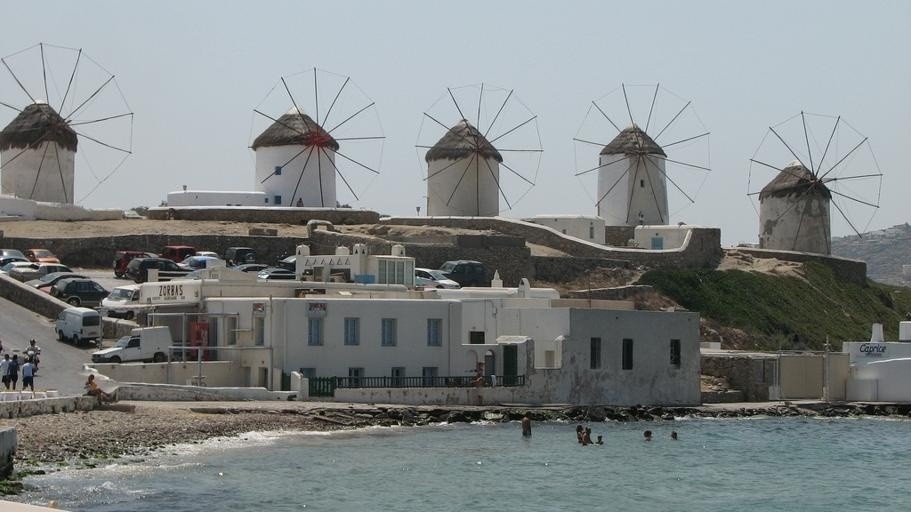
[523,410,532,436]
[577,425,588,443]
[595,434,605,445]
[642,429,654,442]
[477,362,485,376]
[84,374,112,406]
[0,337,42,392]
[582,426,594,446]
[670,430,678,439]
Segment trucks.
[92,325,172,362]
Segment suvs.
[415,259,485,289]
[113,243,346,298]
[0,249,159,320]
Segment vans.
[54,307,104,346]
